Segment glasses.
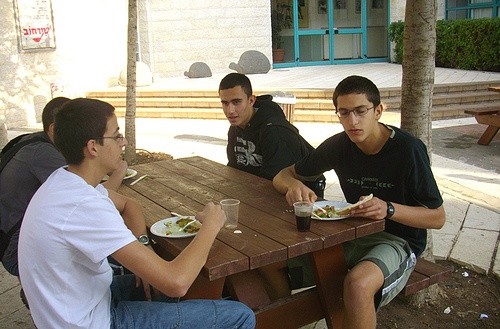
[335,105,374,118]
[89,133,124,142]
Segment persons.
[18,99,256,329]
[273,75,446,329]
[0,97,70,329]
[218,73,326,310]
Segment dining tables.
[103,156,385,329]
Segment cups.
[293,201,314,231]
[219,199,240,229]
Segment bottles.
[315,179,325,194]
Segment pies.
[335,193,373,215]
[177,218,202,233]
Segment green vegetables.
[313,204,336,218]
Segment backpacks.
[260,121,326,198]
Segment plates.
[310,200,355,220]
[150,216,203,238]
[101,169,137,181]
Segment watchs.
[138,234,150,246]
[385,200,396,220]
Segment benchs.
[402,257,452,297]
[464,106,500,145]
